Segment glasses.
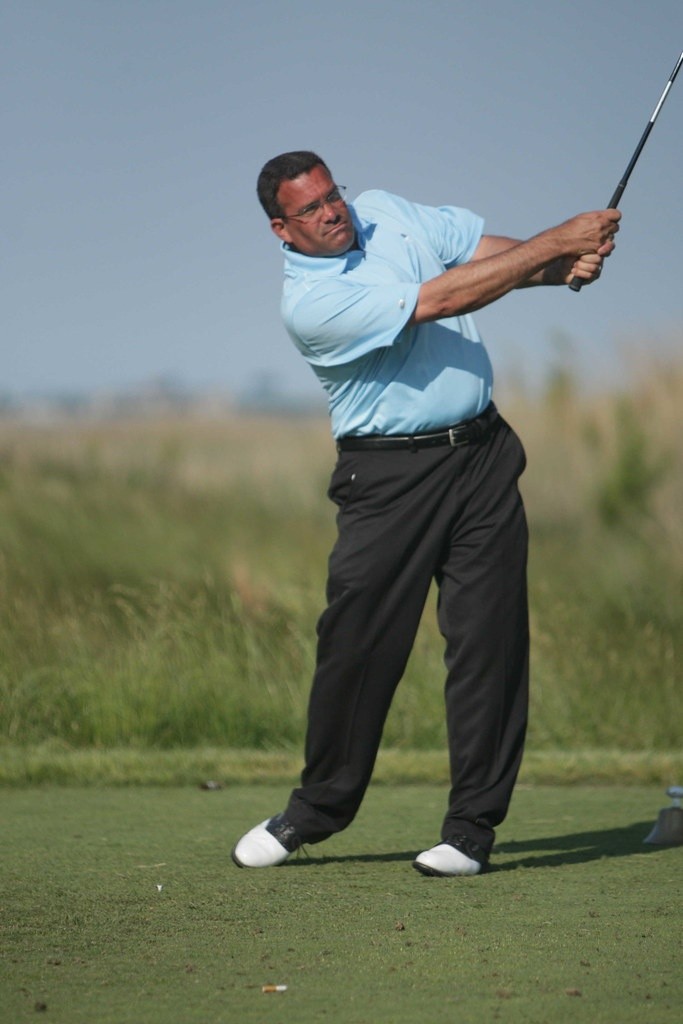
[282,185,347,218]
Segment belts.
[339,399,499,451]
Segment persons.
[232,151,622,874]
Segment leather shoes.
[230,812,310,869]
[411,835,490,878]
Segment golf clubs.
[566,50,683,292]
